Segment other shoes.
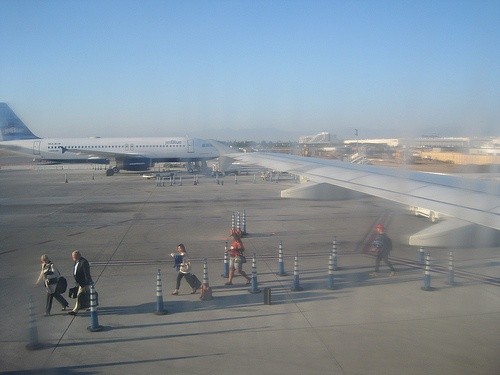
[370,272,380,277]
[225,281,232,285]
[171,292,178,295]
[86,309,90,311]
[390,271,395,276]
[67,310,77,316]
[191,287,197,294]
[247,277,251,285]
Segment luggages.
[190,273,201,288]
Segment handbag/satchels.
[55,277,67,294]
[68,284,79,299]
[77,290,98,309]
[235,249,246,263]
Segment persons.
[170,244,196,294]
[34,255,69,316]
[225,229,251,285]
[68,250,92,315]
[368,224,395,277]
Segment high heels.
[44,313,50,317]
[62,303,68,311]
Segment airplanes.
[0,102,220,177]
[223,150,500,249]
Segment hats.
[376,224,383,229]
